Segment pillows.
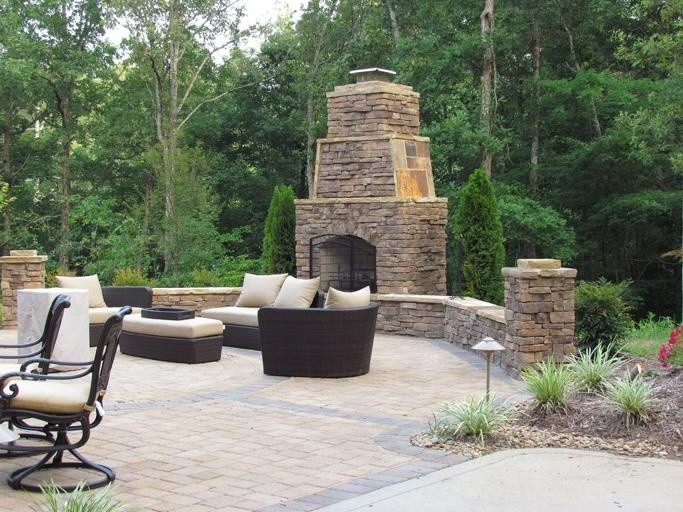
[272,275,319,308]
[234,272,287,307]
[324,285,370,308]
[57,274,107,309]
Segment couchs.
[202,272,324,351]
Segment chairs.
[1,294,71,458]
[258,284,379,377]
[54,273,152,346]
[0,305,132,492]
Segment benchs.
[119,313,224,363]
